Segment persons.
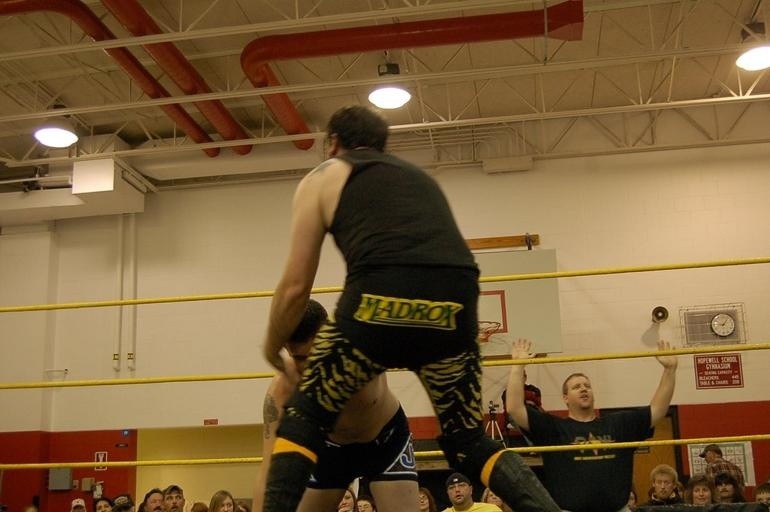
[506,338,681,511]
[248,298,422,511]
[338,473,508,511]
[67,481,247,511]
[259,104,564,511]
[624,439,770,511]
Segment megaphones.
[652,307,668,323]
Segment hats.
[698,444,721,457]
[71,498,86,510]
[111,495,134,512]
[444,472,470,486]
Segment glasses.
[115,503,131,511]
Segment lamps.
[33,117,78,150]
[736,35,770,72]
[367,72,413,110]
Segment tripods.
[485,410,507,449]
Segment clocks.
[712,314,735,337]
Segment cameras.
[488,400,499,409]
